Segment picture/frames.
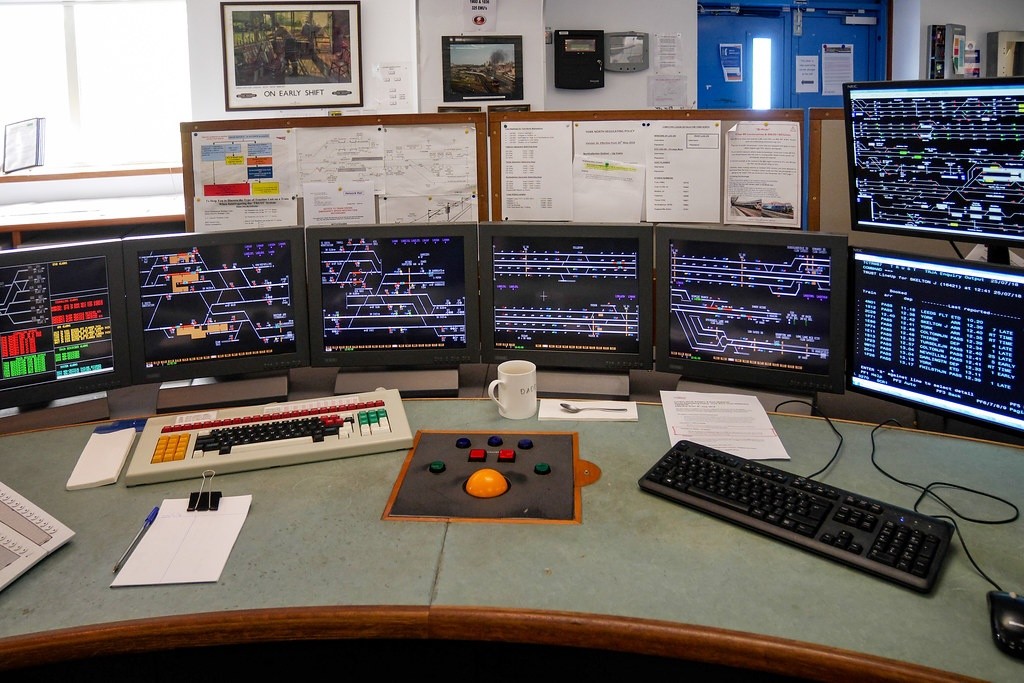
[488,103,530,136]
[438,106,481,112]
[220,1,363,112]
[441,35,523,101]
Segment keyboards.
[638,440,956,594]
[125,388,413,486]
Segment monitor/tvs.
[0,223,1024,433]
[843,76,1024,249]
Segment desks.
[1,379,502,672]
[434,380,1023,683]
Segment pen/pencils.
[111,506,160,573]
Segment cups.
[487,360,537,420]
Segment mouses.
[986,590,1024,663]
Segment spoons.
[560,403,627,413]
